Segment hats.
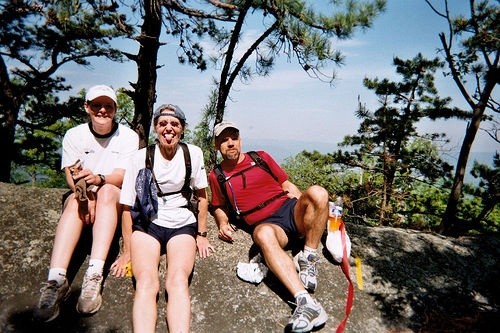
[85,85,117,107]
[212,122,240,138]
[153,104,186,127]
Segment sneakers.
[35,272,103,325]
[282,295,330,333]
[293,247,320,293]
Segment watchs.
[97,174,106,185]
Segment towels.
[325,220,352,263]
[71,167,99,201]
[237,262,269,284]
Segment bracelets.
[196,231,207,236]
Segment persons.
[208,122,329,333]
[109,104,216,333]
[32,84,140,322]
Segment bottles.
[328,197,343,232]
